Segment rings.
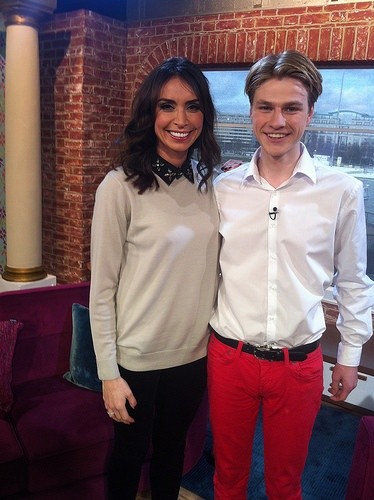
[107,411,115,415]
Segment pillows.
[62,302,103,393]
[0,320,24,409]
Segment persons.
[89,56,220,500]
[207,50,374,500]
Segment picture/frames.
[188,59,373,314]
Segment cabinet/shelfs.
[321,324,373,415]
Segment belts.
[213,331,321,363]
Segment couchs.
[0,282,211,500]
[346,416,374,500]
